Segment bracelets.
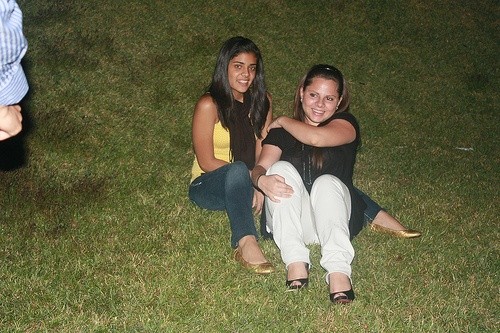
[256,173,264,188]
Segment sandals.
[286,263,310,289]
[328,276,356,304]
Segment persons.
[0,0,29,140]
[251,63,361,303]
[189,35,422,276]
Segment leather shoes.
[366,222,422,238]
[229,245,276,276]
